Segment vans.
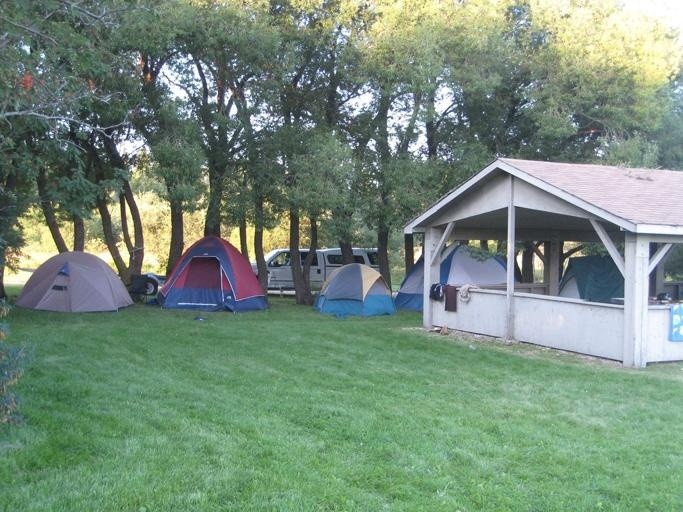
[248,245,380,293]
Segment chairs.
[284,255,291,266]
[125,274,149,304]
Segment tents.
[558,255,624,303]
[394,243,521,312]
[13,250,135,314]
[314,263,396,318]
[156,236,266,312]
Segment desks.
[611,297,682,306]
